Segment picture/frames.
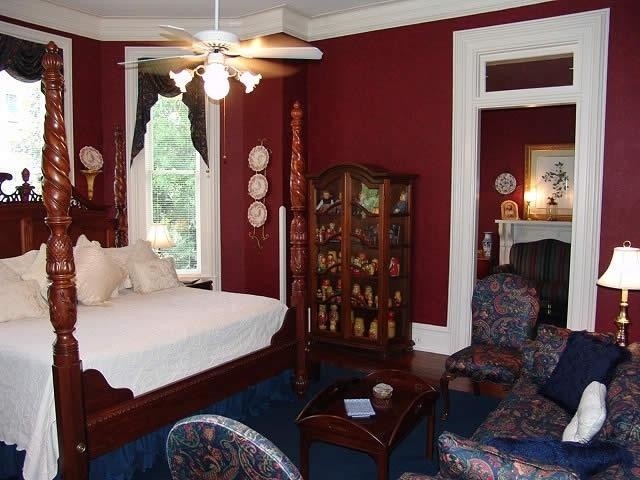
[524,144,575,221]
[501,200,518,220]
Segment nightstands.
[178,276,213,290]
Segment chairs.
[440,272,540,423]
[165,414,303,480]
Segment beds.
[0,39,306,480]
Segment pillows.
[103,239,152,289]
[0,249,40,278]
[488,432,637,477]
[561,381,608,443]
[438,430,580,480]
[533,323,612,386]
[126,257,179,294]
[546,332,626,414]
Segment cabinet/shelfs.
[305,161,418,359]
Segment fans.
[116,1,324,62]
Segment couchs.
[495,238,571,325]
[398,323,640,480]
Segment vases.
[481,231,493,258]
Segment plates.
[248,145,270,172]
[495,173,516,195]
[79,146,104,170]
[247,201,268,228]
[247,174,269,199]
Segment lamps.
[596,240,640,347]
[21,242,50,301]
[72,234,126,307]
[146,223,177,256]
[169,52,262,101]
[0,278,49,322]
